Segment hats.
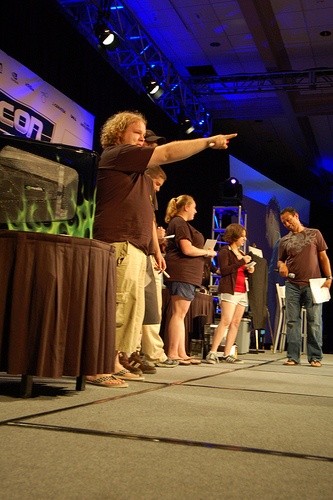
[143,129,163,142]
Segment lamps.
[143,75,159,94]
[93,19,120,50]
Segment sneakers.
[118,352,158,375]
[205,353,244,366]
[151,359,178,368]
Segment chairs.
[273,283,307,354]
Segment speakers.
[192,315,211,360]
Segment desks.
[161,289,212,353]
[1,229,116,395]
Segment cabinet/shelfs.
[210,205,247,285]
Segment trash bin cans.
[236,318,252,354]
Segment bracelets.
[205,249,208,256]
[326,275,333,280]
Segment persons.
[204,222,254,365]
[83,110,238,388]
[277,206,333,366]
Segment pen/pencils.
[164,235,175,239]
[155,263,170,279]
[208,140,229,148]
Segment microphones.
[274,269,295,278]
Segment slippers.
[173,357,203,366]
[283,359,322,367]
[84,368,145,388]
